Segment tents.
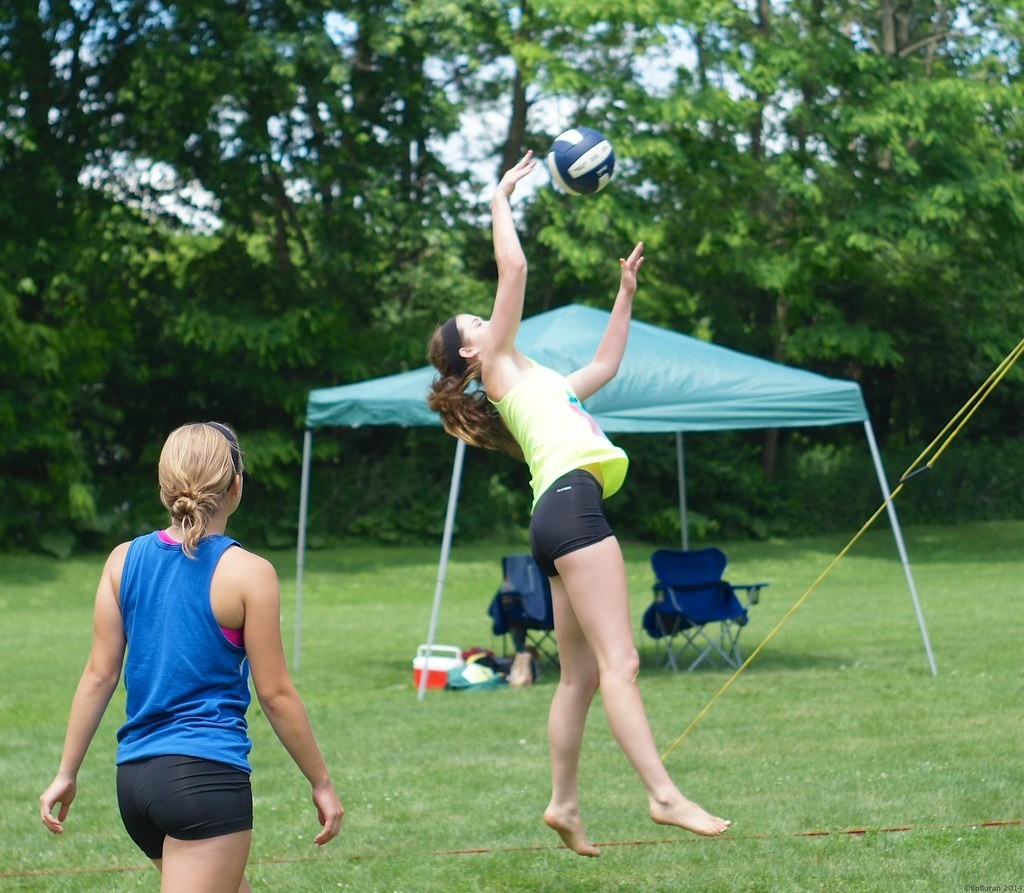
[290,303,943,681]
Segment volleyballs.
[548,126,614,196]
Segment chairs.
[486,555,562,679]
[642,547,769,673]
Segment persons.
[38,421,347,893]
[429,150,733,857]
